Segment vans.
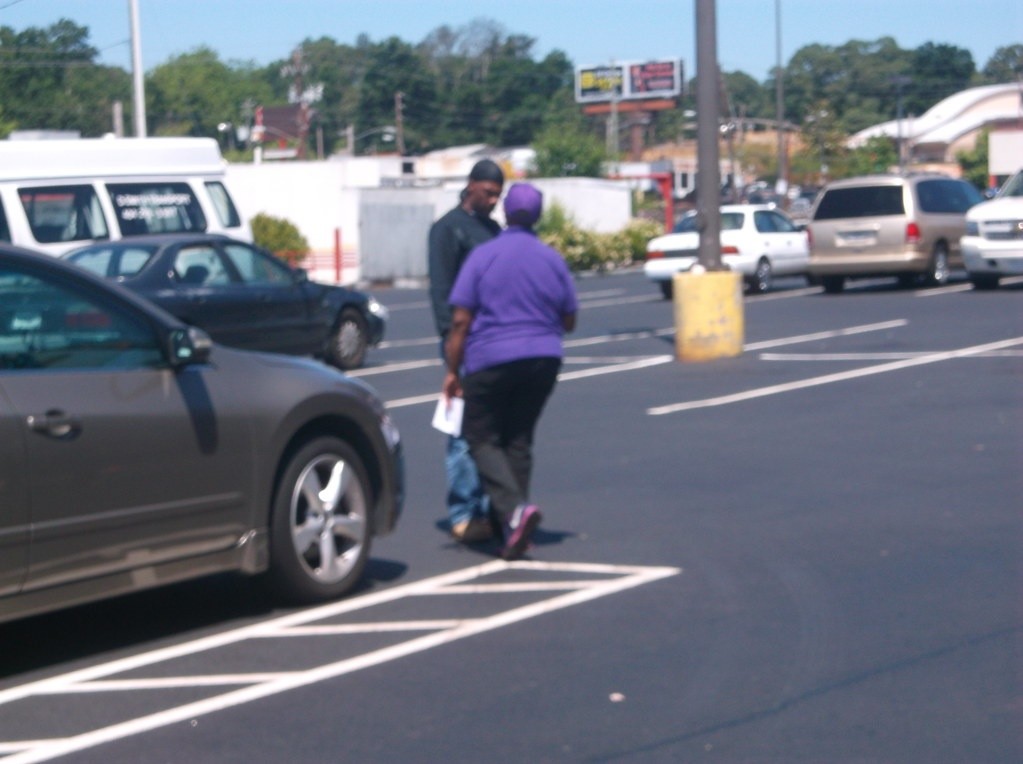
[0,133,258,283]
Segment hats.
[460,160,504,201]
[504,184,543,223]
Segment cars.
[641,202,810,301]
[1,240,407,624]
[14,232,392,372]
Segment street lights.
[345,124,397,157]
[673,109,696,198]
[252,121,324,160]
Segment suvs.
[957,166,1023,290]
[805,174,987,294]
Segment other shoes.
[454,516,495,541]
[499,504,540,560]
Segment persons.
[441,182,578,559]
[425,158,508,540]
